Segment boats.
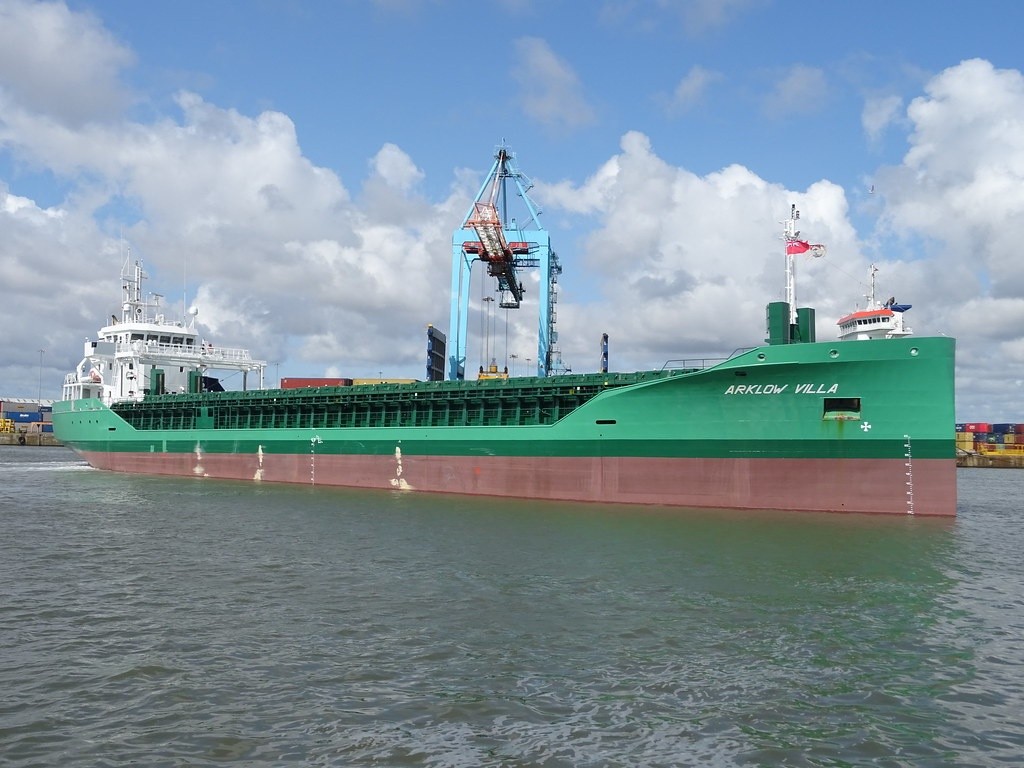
[52,135,959,520]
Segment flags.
[787,239,810,254]
